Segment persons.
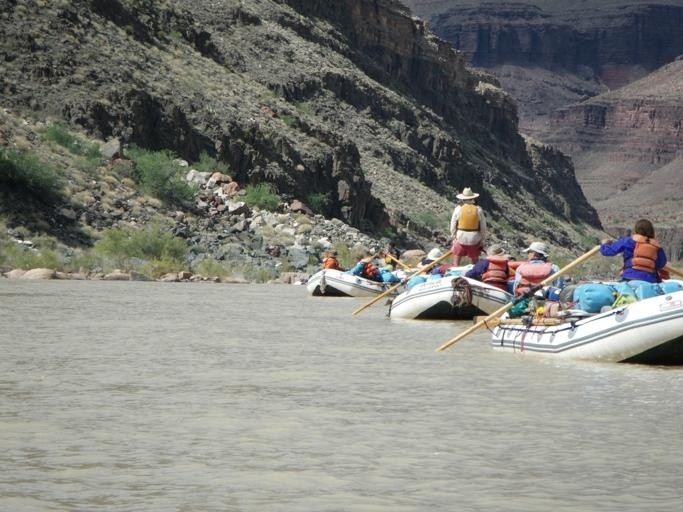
[416,248,444,276]
[598,217,668,282]
[322,240,401,282]
[464,242,509,291]
[512,241,563,299]
[450,186,485,267]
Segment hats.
[521,241,551,258]
[486,244,505,256]
[455,186,480,200]
[328,250,339,258]
[427,247,443,261]
